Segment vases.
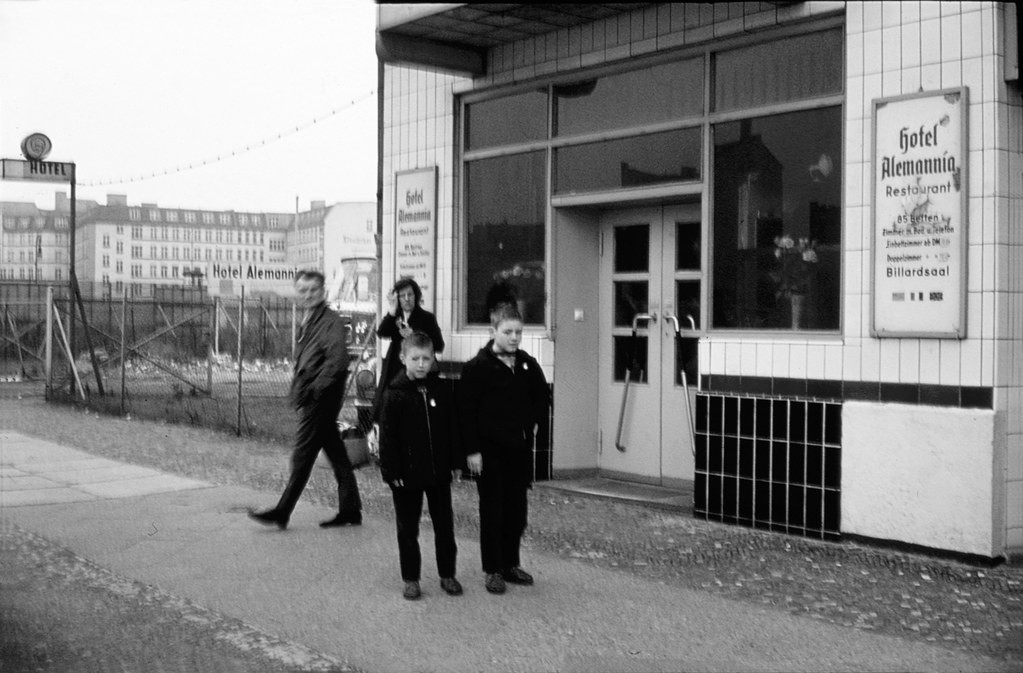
[792,294,804,330]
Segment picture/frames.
[867,87,969,340]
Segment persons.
[379,333,462,599]
[373,278,444,467]
[457,305,548,594]
[249,270,362,529]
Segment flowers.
[769,235,819,301]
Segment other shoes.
[249,508,291,530]
[484,569,506,594]
[500,566,534,586]
[319,510,363,528]
[440,576,463,595]
[403,581,421,599]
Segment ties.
[295,326,302,342]
